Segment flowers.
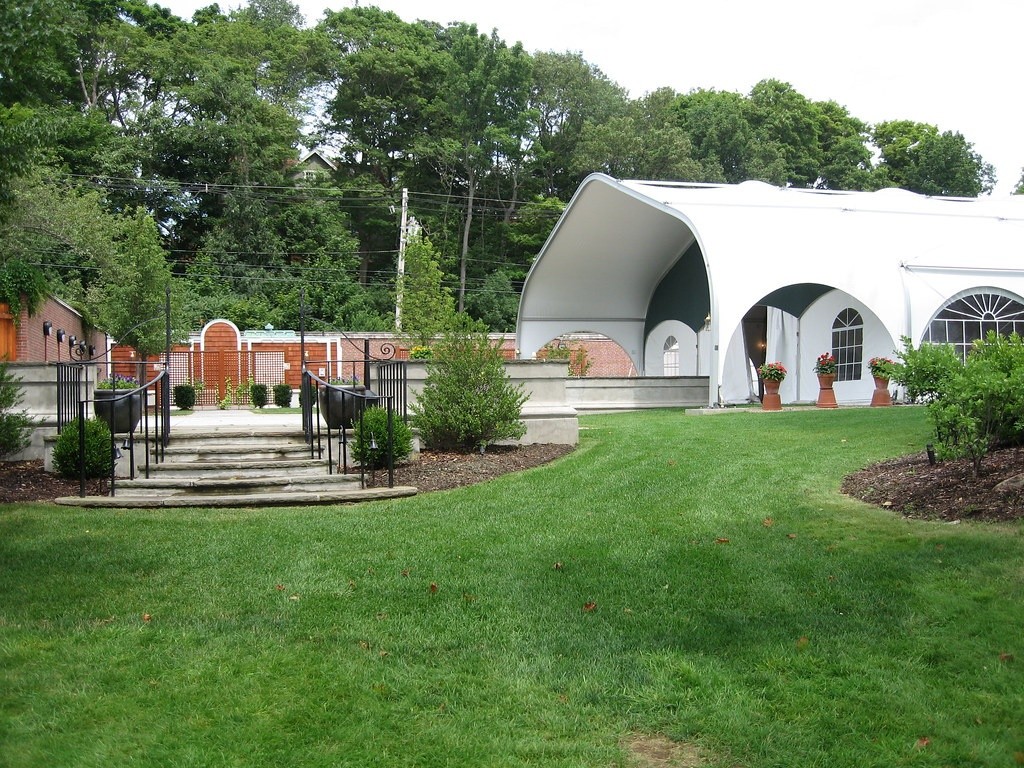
[327,374,360,385]
[97,374,145,389]
[812,352,837,374]
[757,361,787,382]
[868,357,893,378]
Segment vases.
[763,380,780,394]
[870,389,892,406]
[874,377,890,389]
[817,374,836,388]
[762,394,781,410]
[93,390,147,433]
[817,389,838,408]
[319,385,366,429]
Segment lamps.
[100,438,123,493]
[57,328,65,343]
[89,345,95,356]
[704,313,711,331]
[80,339,86,352]
[339,425,348,471]
[43,320,52,336]
[121,439,133,480]
[69,335,77,347]
[369,432,379,487]
